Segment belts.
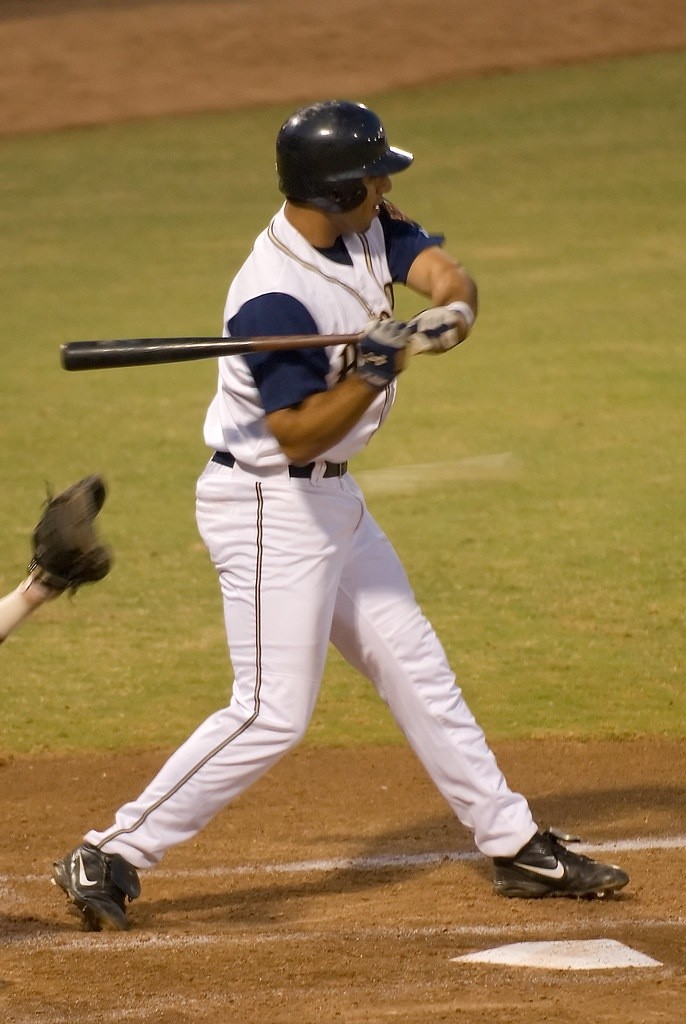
[211,450,347,479]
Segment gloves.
[354,319,414,387]
[407,302,475,354]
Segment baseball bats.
[60,322,441,375]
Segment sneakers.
[51,841,141,931]
[491,830,630,898]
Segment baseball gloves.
[30,472,113,604]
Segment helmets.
[276,100,414,212]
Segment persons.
[0,476,112,643]
[52,100,629,929]
